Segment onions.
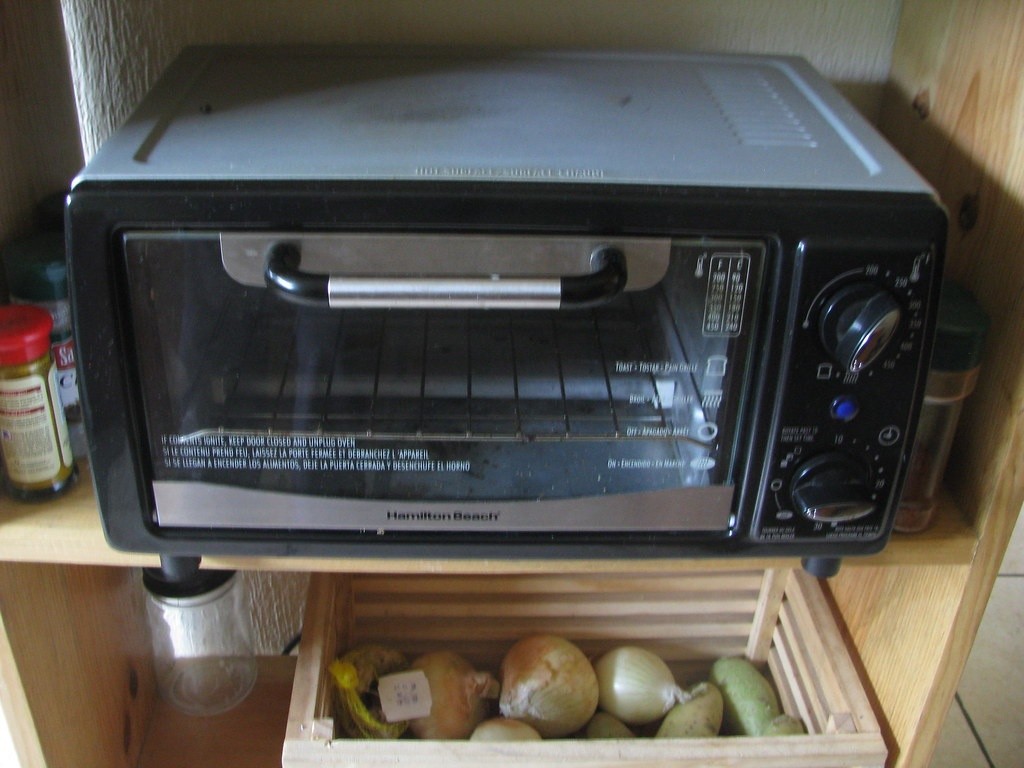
[395,634,708,740]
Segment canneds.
[0,303,79,502]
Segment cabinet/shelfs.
[0,0,1024,768]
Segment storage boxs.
[280,569,890,768]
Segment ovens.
[64,43,951,578]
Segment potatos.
[581,653,807,739]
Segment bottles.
[31,264,88,459]
[892,344,981,532]
[0,304,80,502]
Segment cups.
[142,568,258,717]
